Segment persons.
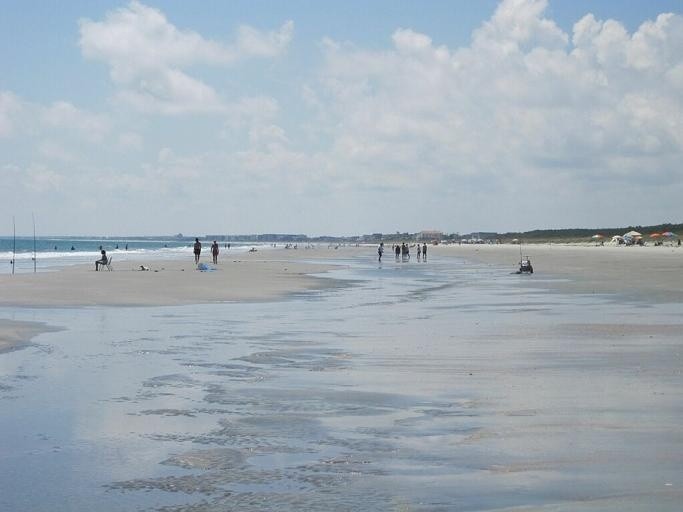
[378,242,384,261]
[194,238,201,261]
[430,238,521,245]
[392,243,427,259]
[95,250,107,271]
[211,241,218,264]
[618,238,664,247]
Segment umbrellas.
[591,230,677,242]
